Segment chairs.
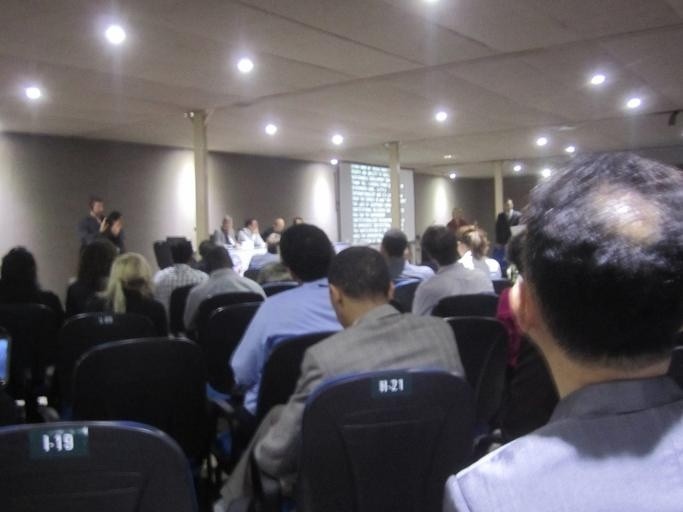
[249,367,467,512]
[46,267,336,512]
[1,421,200,512]
[389,276,420,308]
[431,277,515,454]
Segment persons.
[0,197,559,510]
[442,151,682,512]
